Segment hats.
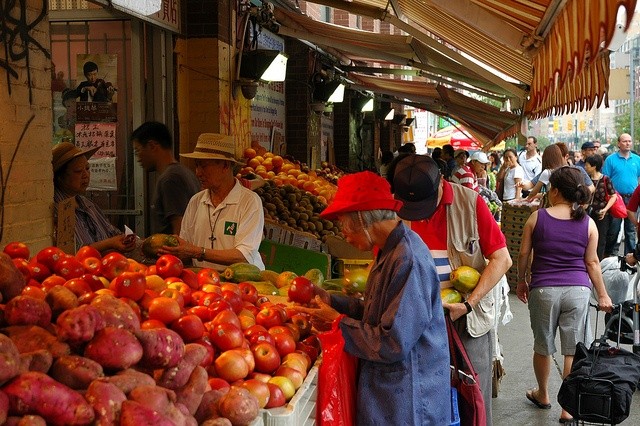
[52,142,102,173]
[392,154,441,221]
[454,149,466,158]
[179,133,247,166]
[471,152,491,165]
[319,170,404,217]
[581,142,599,148]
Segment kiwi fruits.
[242,172,348,244]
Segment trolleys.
[591,255,640,356]
[564,303,622,426]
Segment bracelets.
[517,275,525,282]
[460,300,472,314]
[197,246,205,262]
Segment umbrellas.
[424,124,506,153]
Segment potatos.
[0,253,26,301]
[0,294,257,426]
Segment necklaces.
[207,204,222,249]
[521,144,565,202]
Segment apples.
[0,240,323,410]
[288,277,314,303]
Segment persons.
[496,149,525,200]
[129,121,200,241]
[515,136,543,180]
[593,137,616,161]
[514,166,616,423]
[625,241,640,266]
[398,142,416,157]
[439,145,456,170]
[489,153,501,174]
[139,132,266,271]
[379,151,394,179]
[514,142,596,210]
[58,88,79,129]
[449,152,491,193]
[451,149,470,178]
[74,61,114,102]
[600,133,640,256]
[626,184,640,235]
[52,141,137,256]
[373,154,513,426]
[567,151,581,165]
[583,153,616,261]
[284,170,452,426]
[575,143,599,167]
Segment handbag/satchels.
[603,190,628,218]
[445,372,487,426]
[538,183,555,209]
[559,341,640,424]
[316,328,360,426]
[585,205,604,216]
